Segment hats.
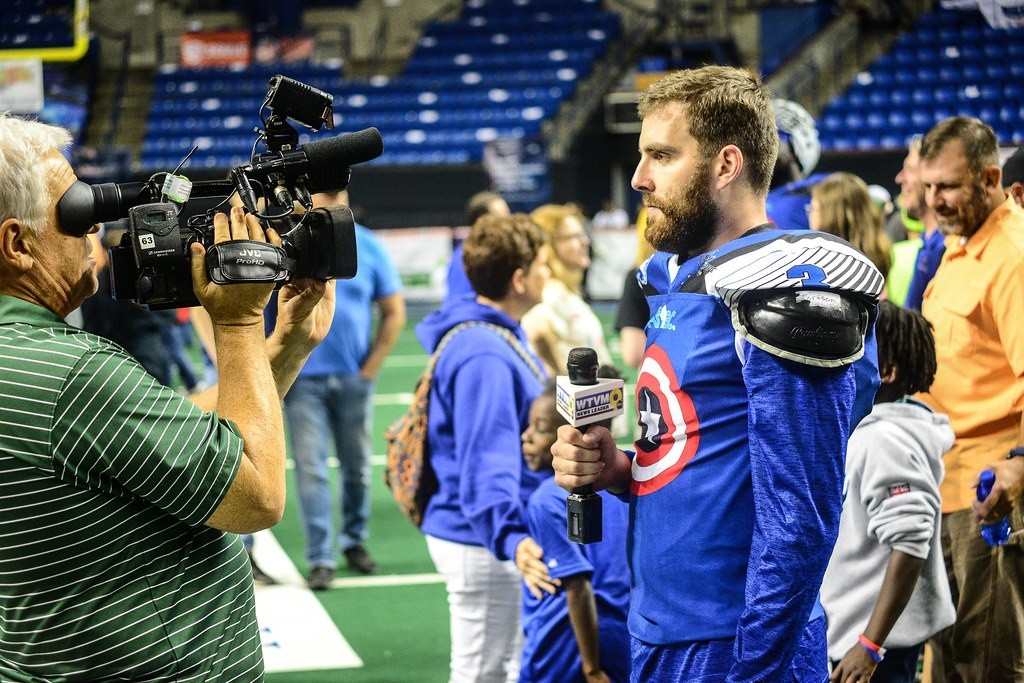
[772,100,820,177]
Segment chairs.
[40,0,625,175]
[815,0,1024,158]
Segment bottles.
[977,468,1011,547]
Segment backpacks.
[386,321,547,526]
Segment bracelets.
[858,633,886,655]
[861,643,884,662]
[1006,447,1024,459]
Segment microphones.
[567,347,603,544]
[230,127,384,178]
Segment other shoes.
[249,554,275,584]
[308,568,333,589]
[343,546,376,574]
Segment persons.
[80,190,406,591]
[446,190,630,683]
[415,213,562,683]
[765,98,1024,683]
[0,116,349,683]
[550,65,886,683]
